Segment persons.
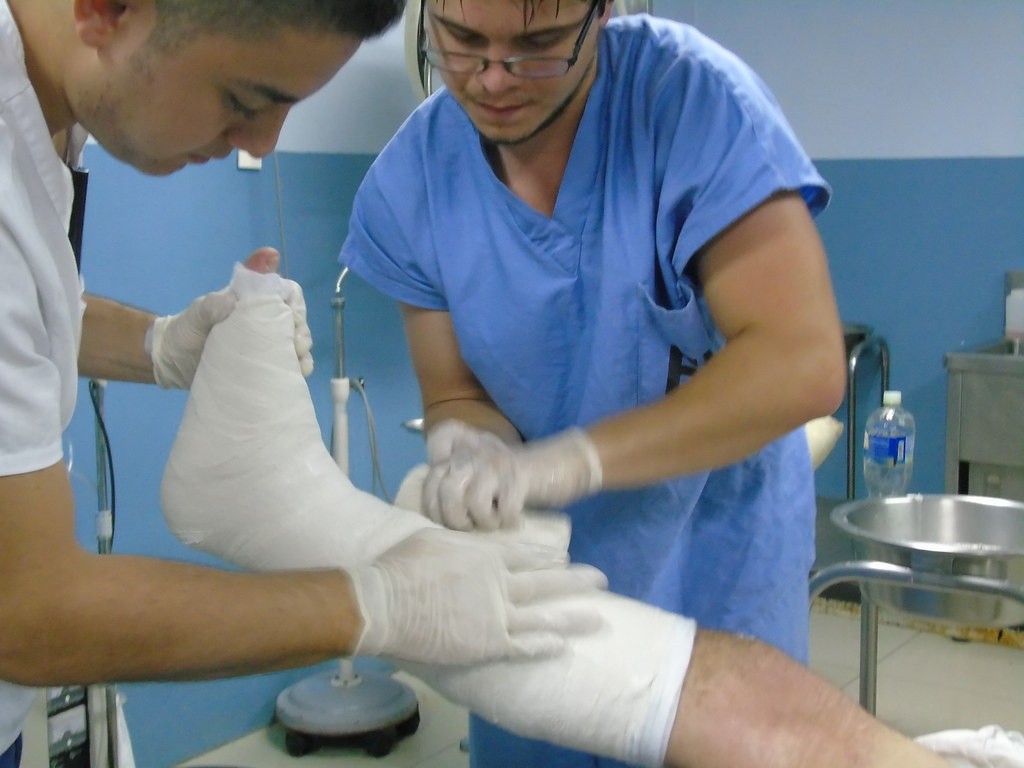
[336,0,846,768]
[162,248,985,767]
[0,1,609,686]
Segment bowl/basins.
[831,495,1024,627]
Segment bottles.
[862,390,916,498]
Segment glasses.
[418,0,602,78]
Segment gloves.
[420,417,506,468]
[424,427,604,532]
[153,277,313,388]
[339,524,608,670]
